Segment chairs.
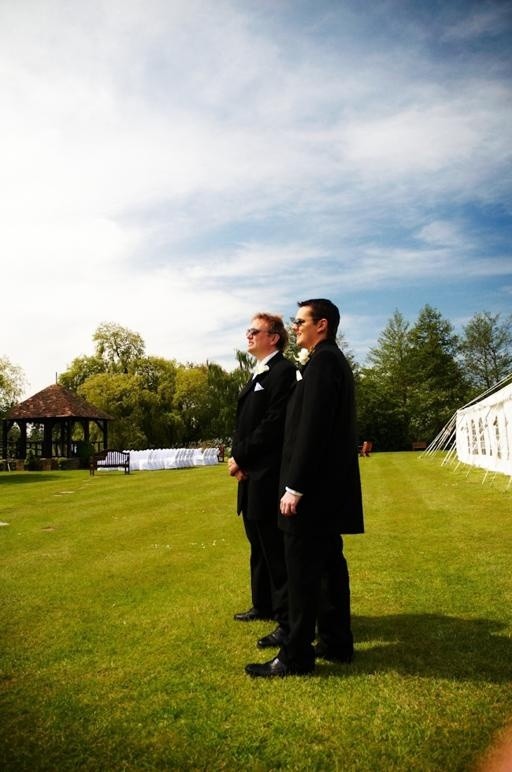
[97,444,225,474]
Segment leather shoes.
[258,626,287,647]
[245,656,294,676]
[234,607,260,620]
[314,642,353,664]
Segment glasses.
[293,320,304,325]
[246,329,260,335]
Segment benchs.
[89,448,130,475]
[412,441,427,450]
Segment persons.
[240,296,369,677]
[227,311,320,650]
